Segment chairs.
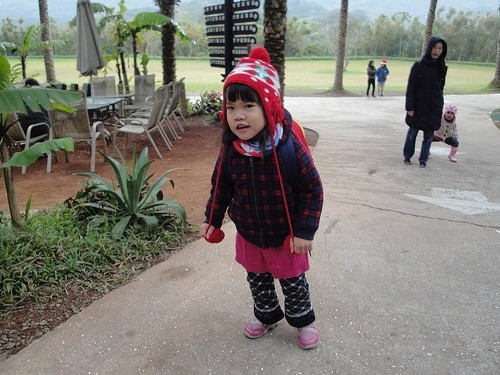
[4,74,185,175]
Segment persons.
[199,48,324,348]
[403,36,448,168]
[17,78,50,142]
[432,104,460,161]
[366,60,377,97]
[375,59,389,96]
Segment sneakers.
[243,318,277,339]
[297,323,318,350]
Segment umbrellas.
[76,0,105,80]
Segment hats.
[443,105,457,123]
[381,59,387,65]
[204,48,294,250]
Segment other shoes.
[449,157,457,162]
[420,163,425,168]
[404,157,410,165]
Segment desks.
[86,92,136,145]
